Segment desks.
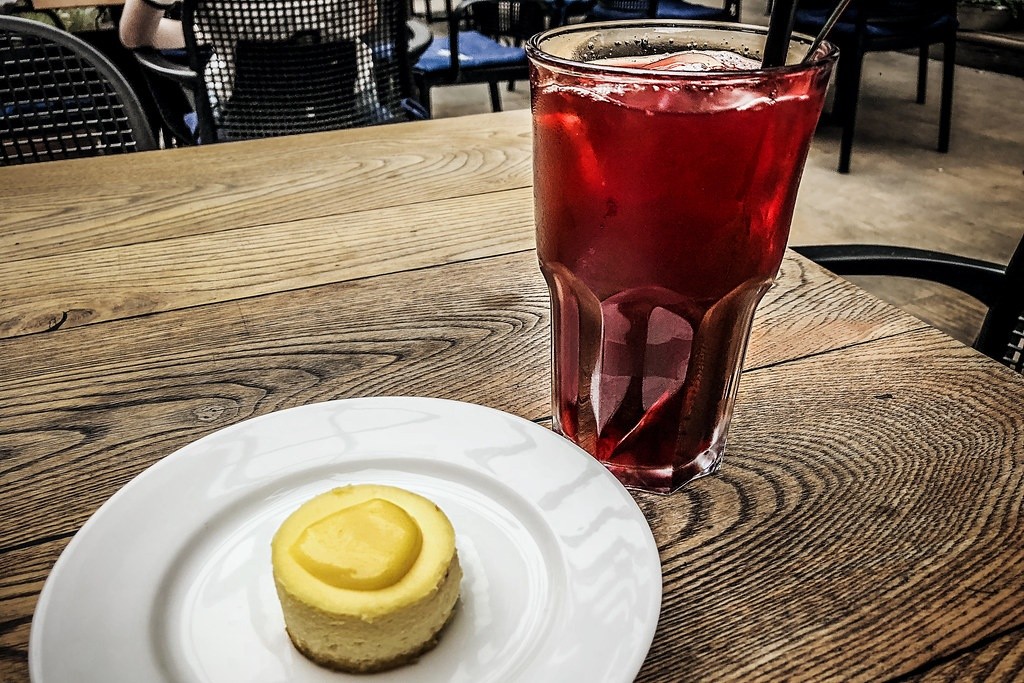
[0,108,1024,683]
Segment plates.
[26,397,663,683]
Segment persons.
[118,0,389,146]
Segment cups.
[525,20,840,498]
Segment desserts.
[272,484,462,674]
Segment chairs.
[788,233,1024,377]
[0,0,962,178]
